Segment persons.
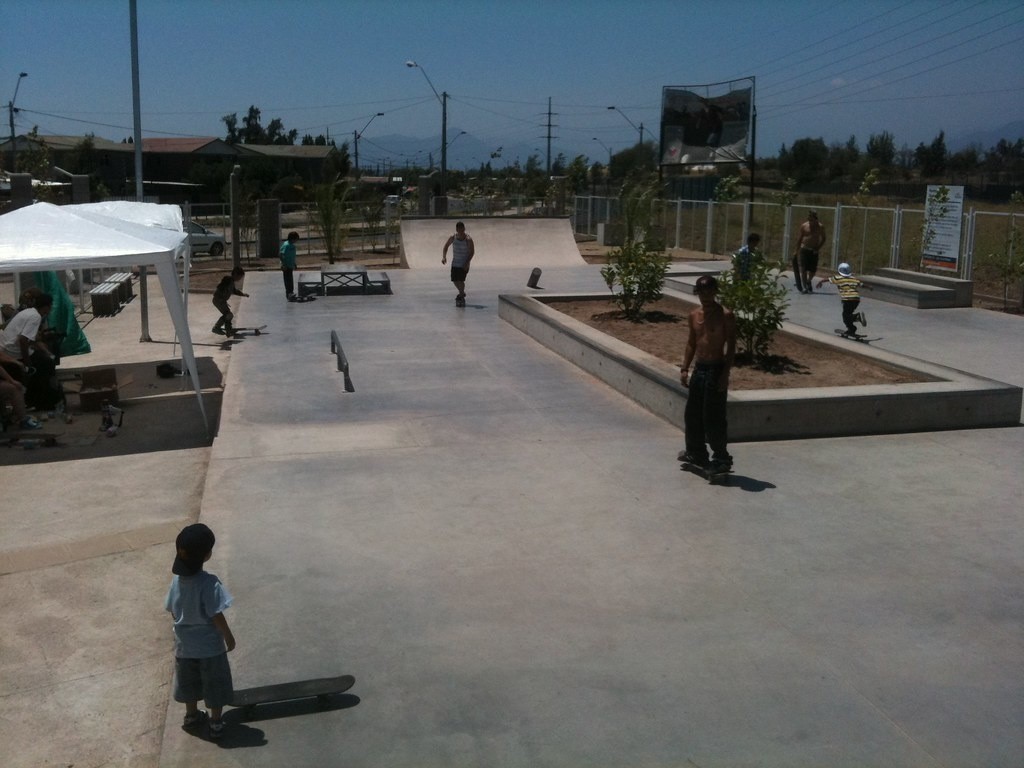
[212,267,249,338]
[816,263,873,334]
[279,231,300,298]
[739,233,761,281]
[794,211,826,293]
[164,525,235,737]
[681,276,735,474]
[442,221,475,298]
[0,288,61,428]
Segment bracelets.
[681,368,689,373]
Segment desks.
[319,262,368,295]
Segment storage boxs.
[78,367,119,411]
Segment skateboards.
[294,292,317,302]
[790,255,803,292]
[0,430,67,448]
[678,449,736,484]
[221,325,268,336]
[225,674,356,716]
[835,328,868,341]
[454,296,466,307]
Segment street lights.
[593,137,612,161]
[406,61,448,196]
[607,105,645,152]
[9,72,27,172]
[354,112,385,180]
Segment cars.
[382,195,402,209]
[178,219,227,256]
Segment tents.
[0,200,209,438]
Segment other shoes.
[678,453,709,465]
[51,397,65,406]
[19,418,43,430]
[210,718,233,736]
[703,460,732,474]
[287,294,297,303]
[212,327,225,335]
[456,294,466,300]
[226,329,238,338]
[803,280,812,294]
[844,330,855,337]
[184,708,208,729]
[856,312,867,327]
[22,400,36,411]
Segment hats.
[838,263,851,277]
[171,523,215,578]
[34,294,53,307]
[693,275,717,293]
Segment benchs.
[364,271,391,295]
[855,267,977,309]
[104,272,134,303]
[297,272,321,297]
[89,282,122,318]
